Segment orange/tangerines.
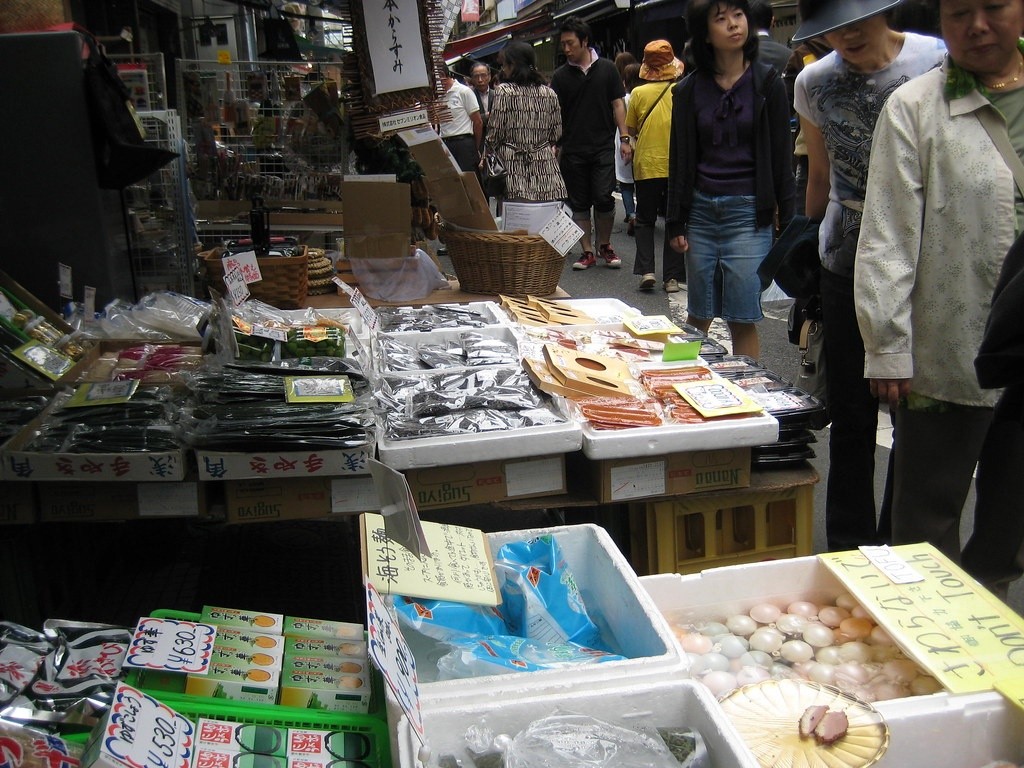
[247,617,362,688]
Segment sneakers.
[572,250,596,269]
[662,279,680,292]
[640,273,656,289]
[596,243,621,268]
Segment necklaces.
[979,47,1022,88]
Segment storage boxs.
[625,478,814,576]
[61,608,395,768]
[340,180,412,260]
[0,273,779,525]
[123,109,196,303]
[396,128,500,233]
[383,524,1023,768]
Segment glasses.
[473,73,490,78]
[501,63,509,72]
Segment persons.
[434,37,566,255]
[549,16,633,270]
[779,0,950,554]
[245,70,272,115]
[846,0,1024,605]
[611,0,791,365]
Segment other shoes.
[627,216,636,237]
[437,246,449,256]
[611,226,622,233]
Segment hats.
[638,39,684,80]
[790,0,903,43]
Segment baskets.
[197,245,308,310]
[58,610,393,768]
[433,211,565,298]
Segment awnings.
[440,13,549,63]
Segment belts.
[442,134,472,143]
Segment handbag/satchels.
[761,280,795,311]
[793,321,831,431]
[478,144,508,196]
[80,33,180,190]
[258,5,305,62]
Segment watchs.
[619,136,630,143]
